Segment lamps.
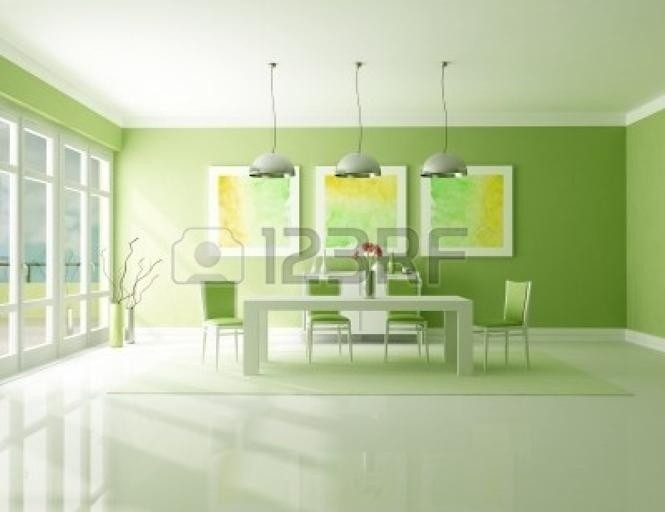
[420,61,469,178]
[335,61,384,178]
[248,63,298,178]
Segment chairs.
[199,281,242,371]
[304,279,354,365]
[474,280,534,372]
[384,277,430,365]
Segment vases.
[363,271,374,297]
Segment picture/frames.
[420,165,514,257]
[314,165,408,257]
[204,166,300,255]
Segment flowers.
[353,243,383,271]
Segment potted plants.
[101,238,164,348]
